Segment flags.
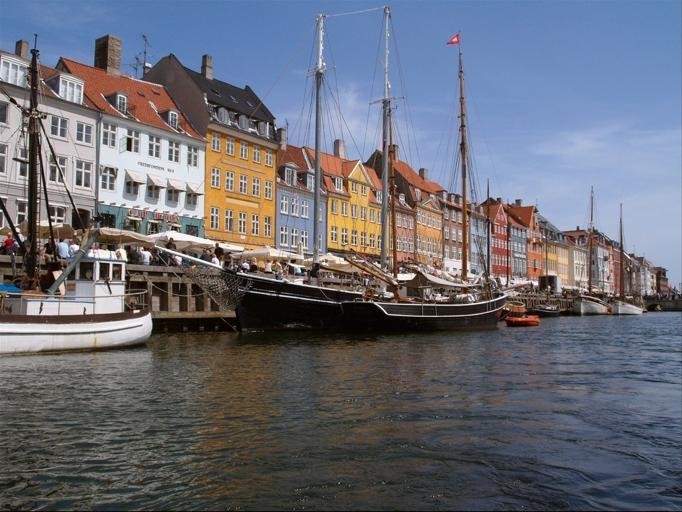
[446,32,460,44]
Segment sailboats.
[532,185,644,315]
[143,6,509,338]
[0,34,154,355]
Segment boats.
[502,300,540,326]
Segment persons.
[321,259,328,278]
[223,251,305,278]
[4,228,80,263]
[88,238,224,268]
